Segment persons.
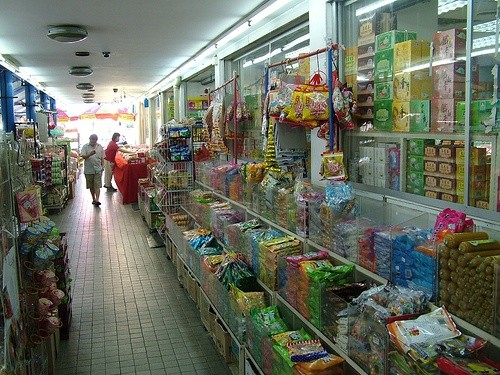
[80,134,106,205]
[103,132,136,192]
[118,135,127,145]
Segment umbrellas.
[49,107,80,122]
[79,104,135,120]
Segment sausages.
[439,230,500,339]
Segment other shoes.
[104,184,111,188]
[108,188,117,192]
[95,202,101,204]
[92,200,96,204]
